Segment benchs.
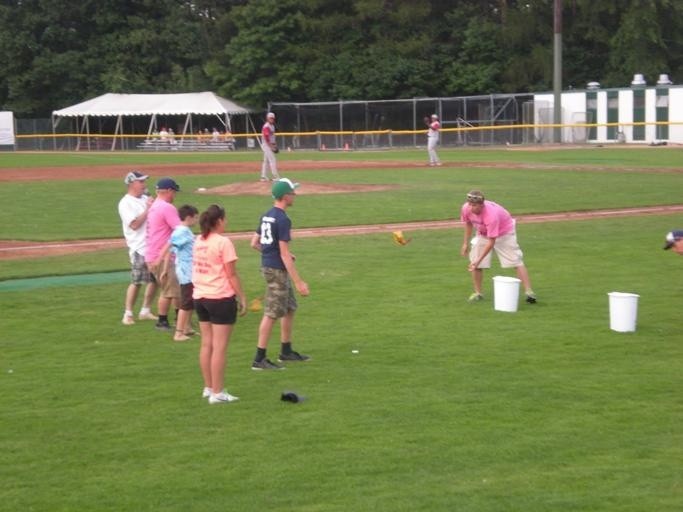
[70,133,233,152]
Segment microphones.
[143,188,152,198]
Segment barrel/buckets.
[492,276,522,312]
[607,292,640,333]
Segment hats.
[661,229,683,251]
[265,112,276,120]
[271,177,301,200]
[155,177,181,192]
[124,171,149,183]
[431,115,438,118]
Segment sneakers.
[467,291,483,303]
[251,357,285,371]
[525,289,537,303]
[201,386,211,398]
[122,314,136,325]
[138,312,159,321]
[278,350,310,363]
[208,391,240,403]
[155,321,175,331]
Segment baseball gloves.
[272,145,278,153]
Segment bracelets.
[463,244,468,250]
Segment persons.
[117,171,159,325]
[461,190,537,304]
[250,177,312,371]
[169,204,201,341]
[662,231,683,255]
[426,114,442,166]
[191,204,247,404]
[259,112,281,182]
[151,127,236,152]
[143,178,182,330]
